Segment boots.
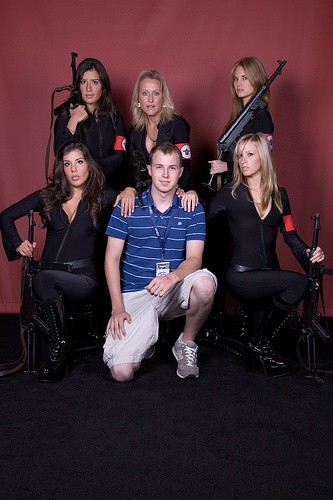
[204,326,249,362]
[206,276,229,326]
[39,291,73,385]
[248,294,299,378]
[68,321,103,367]
[238,301,250,330]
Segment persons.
[53,57,274,340]
[0,138,139,383]
[181,133,325,378]
[103,141,218,380]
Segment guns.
[18,209,52,378]
[134,149,147,181]
[286,212,333,387]
[200,57,288,194]
[53,50,87,144]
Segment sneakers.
[172,331,201,380]
[144,345,156,360]
